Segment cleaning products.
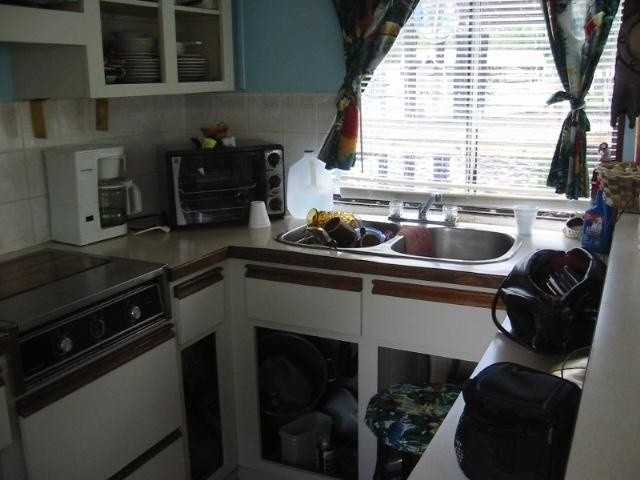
[580,178,613,255]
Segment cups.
[321,215,357,247]
[389,198,404,218]
[442,203,463,222]
[513,202,539,236]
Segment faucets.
[419,191,436,221]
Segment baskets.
[597,161,639,210]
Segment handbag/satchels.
[492,247,607,356]
[453,361,581,480]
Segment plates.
[103,26,210,83]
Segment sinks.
[274,218,400,253]
[391,221,525,264]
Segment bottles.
[305,207,360,232]
[313,437,335,474]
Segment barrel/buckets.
[285,150,333,218]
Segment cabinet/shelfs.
[366,271,506,478]
[0,0,234,100]
[7,319,191,478]
[170,263,238,479]
[242,261,365,479]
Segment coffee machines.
[41,142,142,247]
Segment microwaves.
[156,139,287,228]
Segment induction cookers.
[1,247,169,325]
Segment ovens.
[15,284,191,479]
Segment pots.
[258,330,340,420]
